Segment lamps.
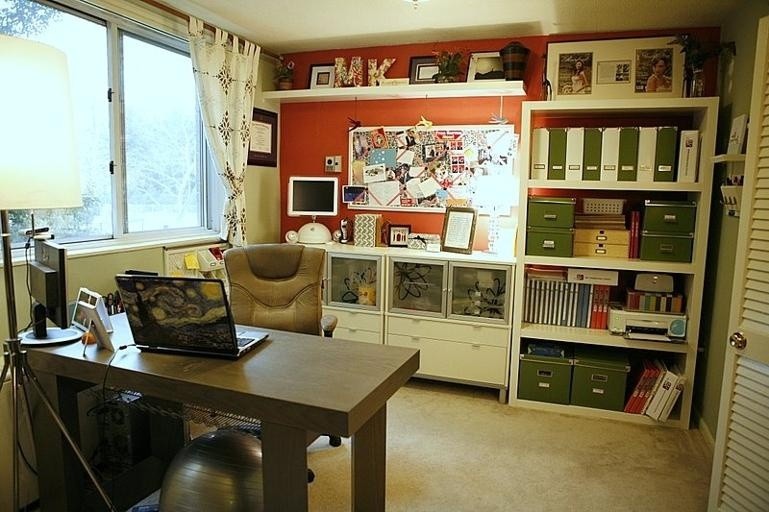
[0,35,128,512]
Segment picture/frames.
[307,63,336,88]
[246,106,279,169]
[727,113,749,155]
[465,51,505,81]
[77,300,114,359]
[408,55,439,83]
[440,206,477,253]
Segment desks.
[24,310,420,512]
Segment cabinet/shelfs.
[506,95,721,431]
[279,240,519,406]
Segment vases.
[279,77,292,89]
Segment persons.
[571,60,588,93]
[427,146,435,157]
[395,164,412,184]
[450,141,464,174]
[365,169,383,177]
[406,129,415,146]
[646,56,671,92]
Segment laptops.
[114,273,269,360]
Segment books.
[575,210,640,258]
[530,126,700,182]
[622,360,684,423]
[524,264,618,330]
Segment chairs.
[221,245,339,484]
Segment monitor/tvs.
[19,239,83,348]
[287,177,338,243]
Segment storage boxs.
[518,343,574,405]
[570,343,630,411]
[525,194,696,263]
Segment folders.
[531,127,677,181]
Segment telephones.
[339,219,349,244]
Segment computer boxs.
[95,396,184,465]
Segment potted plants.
[667,30,719,98]
[435,49,461,83]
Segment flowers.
[275,60,296,77]
